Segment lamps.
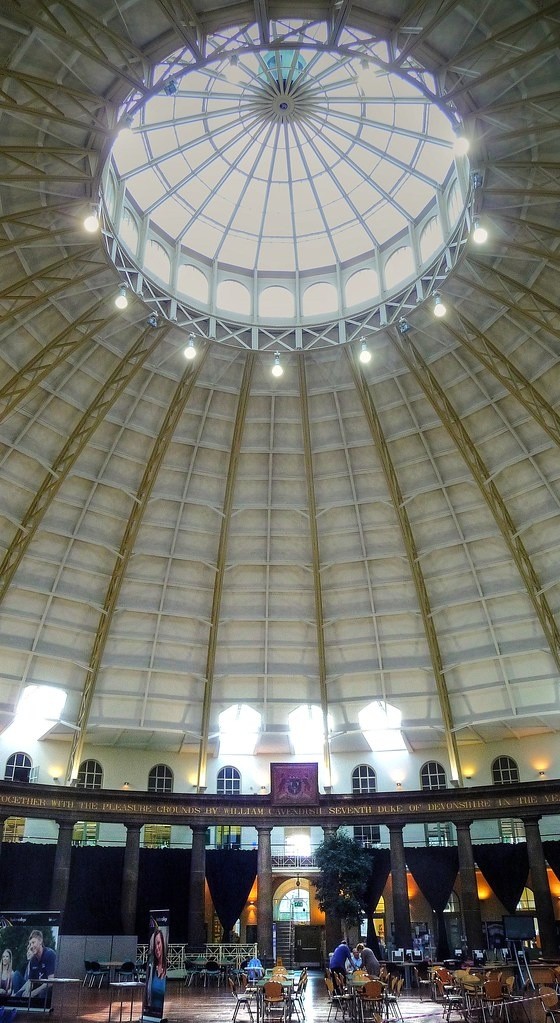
[466,777,471,779]
[53,777,58,780]
[397,783,401,786]
[540,772,544,775]
[124,782,129,785]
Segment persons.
[240,956,251,970]
[145,929,167,1014]
[0,930,55,997]
[330,939,381,1006]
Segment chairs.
[183,960,308,1023]
[324,964,406,1023]
[83,960,148,991]
[413,967,560,1023]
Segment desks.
[98,962,125,983]
[256,980,293,1021]
[28,978,83,1023]
[244,966,263,987]
[265,969,294,975]
[109,981,147,1023]
[349,981,388,1022]
[191,961,235,986]
[262,974,295,994]
